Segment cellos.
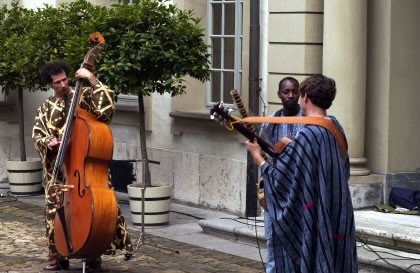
[48,32,117,273]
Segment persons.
[245,76,359,273]
[257,77,304,273]
[32,62,134,273]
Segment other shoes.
[44,260,69,270]
[85,261,101,273]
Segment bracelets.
[260,161,266,168]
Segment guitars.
[209,102,294,163]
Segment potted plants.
[0,0,213,228]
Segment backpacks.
[388,187,420,211]
